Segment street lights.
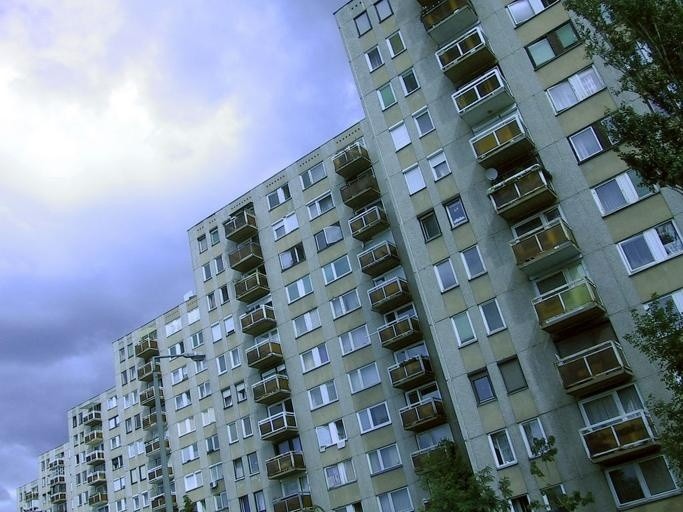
[152,352,206,512]
